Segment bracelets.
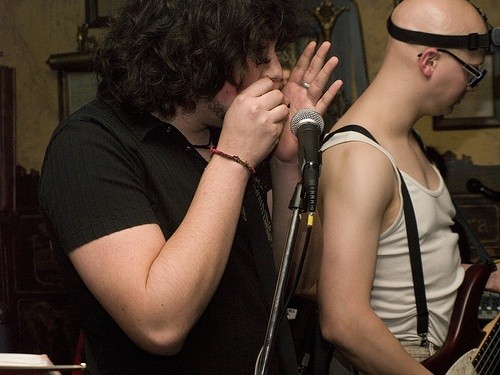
[207,145,254,173]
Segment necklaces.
[192,137,212,149]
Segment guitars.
[275,292,336,375]
[420,262,500,375]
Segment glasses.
[417,48,487,88]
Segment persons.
[314,0,500,375]
[37,1,343,375]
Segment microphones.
[465,178,500,204]
[289,108,325,211]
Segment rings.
[299,80,310,88]
